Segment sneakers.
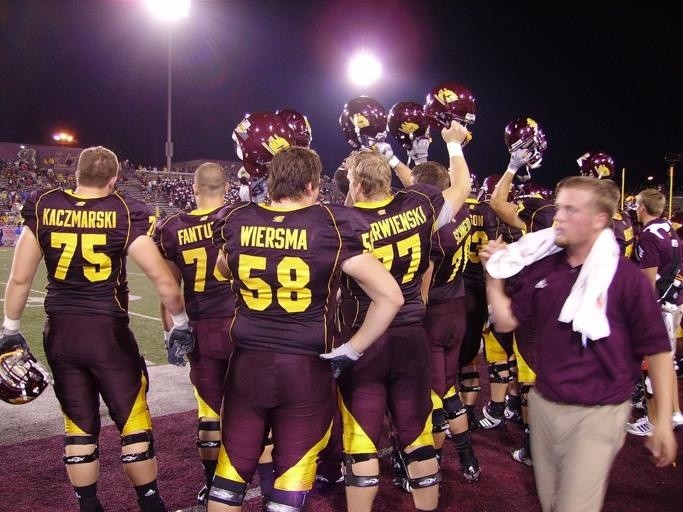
[629,382,648,410]
[471,405,507,431]
[624,416,658,437]
[197,485,215,503]
[508,439,539,468]
[499,394,524,424]
[670,414,683,432]
[462,460,482,485]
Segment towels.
[483,223,621,349]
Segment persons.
[372,140,483,495]
[0,149,196,511]
[153,163,278,512]
[216,158,245,204]
[316,172,346,205]
[602,179,635,265]
[1,144,78,241]
[341,121,471,512]
[375,140,415,190]
[402,138,508,433]
[212,146,405,512]
[478,177,677,512]
[474,174,523,430]
[490,162,559,468]
[624,188,683,436]
[115,156,196,223]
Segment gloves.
[319,344,363,380]
[507,149,532,172]
[372,141,400,168]
[169,324,196,358]
[165,341,187,368]
[10,334,28,352]
[406,138,430,166]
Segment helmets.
[231,111,297,179]
[577,152,616,180]
[277,110,311,149]
[339,97,388,149]
[386,101,431,144]
[524,182,554,199]
[1,348,49,406]
[423,83,475,147]
[479,174,516,200]
[334,159,351,196]
[503,115,546,166]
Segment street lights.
[143,0,192,168]
